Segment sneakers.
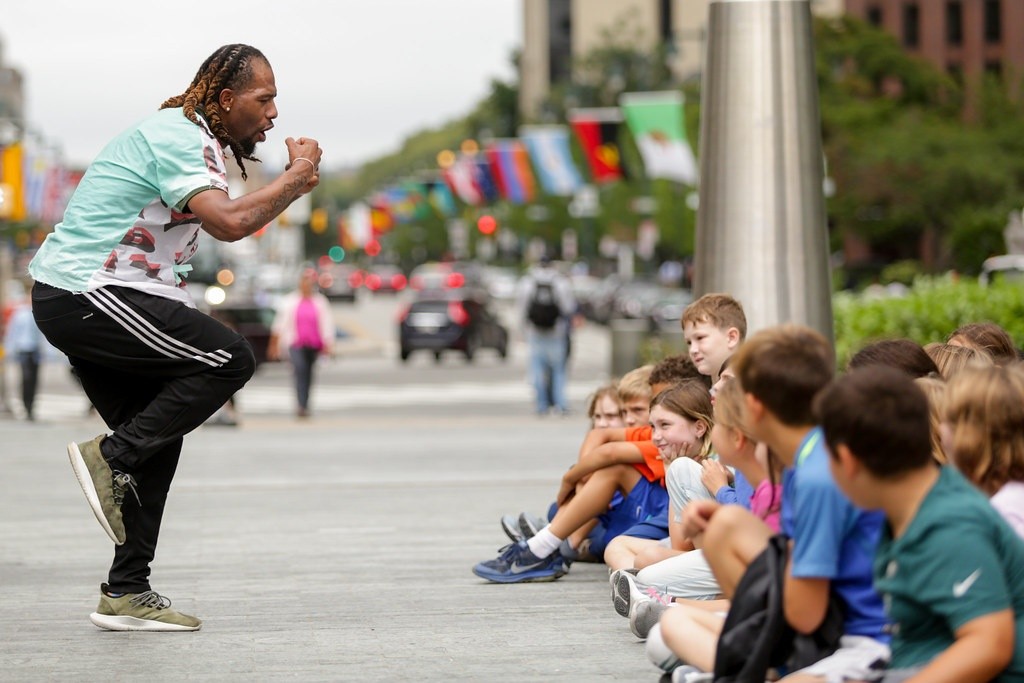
[91,583,202,632]
[67,433,143,546]
[500,513,569,574]
[472,540,566,581]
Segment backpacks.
[527,272,562,331]
[710,536,838,683]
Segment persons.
[7,304,40,420]
[682,295,747,387]
[288,270,334,417]
[732,327,891,683]
[848,324,1024,464]
[820,365,1024,683]
[203,397,238,427]
[515,253,574,411]
[473,357,783,682]
[938,365,1024,537]
[29,44,323,632]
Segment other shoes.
[609,571,638,618]
[628,598,668,638]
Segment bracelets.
[293,158,314,177]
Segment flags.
[569,107,632,182]
[619,90,697,185]
[518,124,586,194]
[337,154,494,252]
[489,142,539,203]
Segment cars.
[368,265,406,293]
[396,298,509,359]
[319,264,357,300]
[410,262,490,306]
